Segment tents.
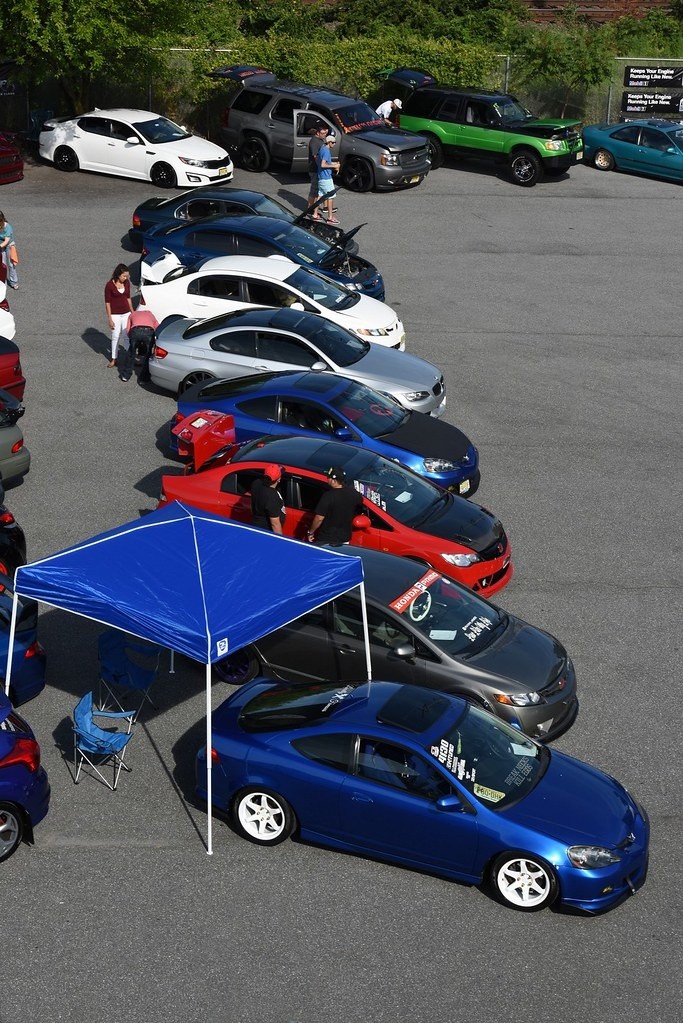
[4,499,372,855]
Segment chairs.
[365,742,412,788]
[71,692,136,791]
[94,630,162,726]
[334,600,387,645]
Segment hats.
[264,464,286,481]
[323,465,347,483]
[394,99,402,109]
[325,135,336,143]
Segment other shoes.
[323,207,338,212]
[307,210,314,215]
[139,379,151,385]
[327,216,340,224]
[108,359,118,368]
[121,374,129,382]
[312,215,323,221]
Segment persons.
[307,126,338,213]
[121,310,160,384]
[0,211,19,290]
[306,466,363,544]
[104,263,134,368]
[250,464,286,535]
[376,99,402,123]
[311,135,340,225]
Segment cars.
[139,212,386,302]
[148,307,447,418]
[127,171,360,256]
[213,544,580,744]
[0,131,24,186]
[581,119,683,185]
[167,369,480,499]
[38,106,234,187]
[193,675,651,918]
[0,207,52,861]
[135,247,407,352]
[156,408,514,600]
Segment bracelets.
[307,531,313,536]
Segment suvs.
[205,64,432,193]
[375,65,584,189]
